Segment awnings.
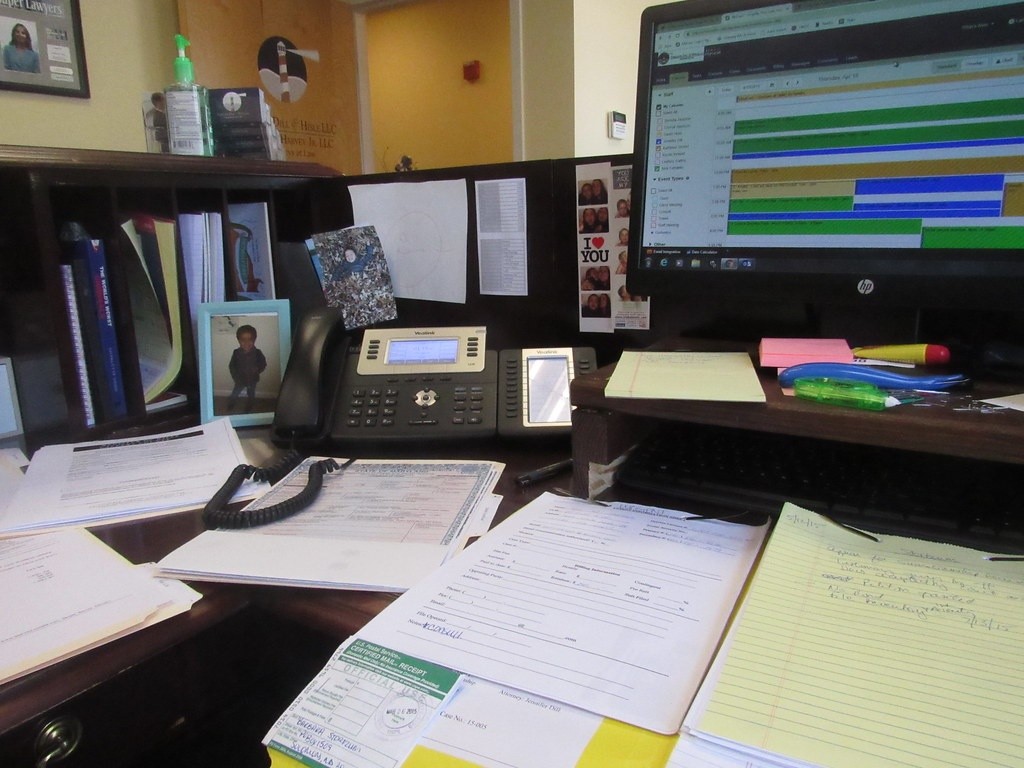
[176,200,279,363]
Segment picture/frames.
[196,298,292,426]
[1,0,93,101]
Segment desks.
[0,405,1024,766]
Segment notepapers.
[760,334,854,367]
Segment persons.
[577,177,648,318]
[227,324,268,415]
[151,92,171,154]
[2,23,42,74]
[326,235,374,281]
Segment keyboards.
[614,420,1024,556]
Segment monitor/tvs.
[626,0,1022,325]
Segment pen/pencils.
[514,457,576,488]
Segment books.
[54,196,182,427]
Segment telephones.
[271,290,603,455]
